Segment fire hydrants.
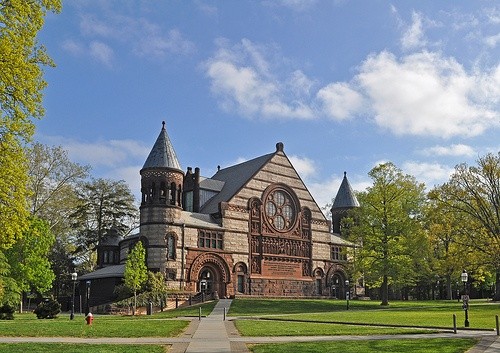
[85,313,93,325]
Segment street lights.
[345,280,350,309]
[70,272,78,319]
[460,269,470,328]
